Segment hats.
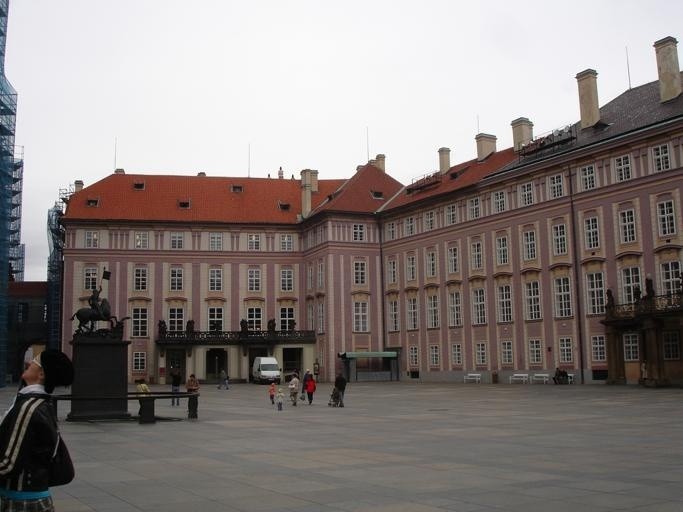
[41,350,75,394]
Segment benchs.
[509,373,574,385]
[463,373,481,384]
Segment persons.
[223,376,229,389]
[168,367,182,407]
[552,367,560,385]
[88,285,106,321]
[274,387,285,411]
[0,345,76,512]
[135,377,150,414]
[559,369,568,385]
[334,373,346,407]
[184,373,201,413]
[267,381,276,406]
[300,368,310,393]
[215,366,227,390]
[640,358,648,380]
[287,372,300,407]
[304,373,316,404]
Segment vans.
[252,357,282,385]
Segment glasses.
[33,356,42,368]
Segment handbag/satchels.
[48,432,74,487]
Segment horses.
[68,298,118,333]
